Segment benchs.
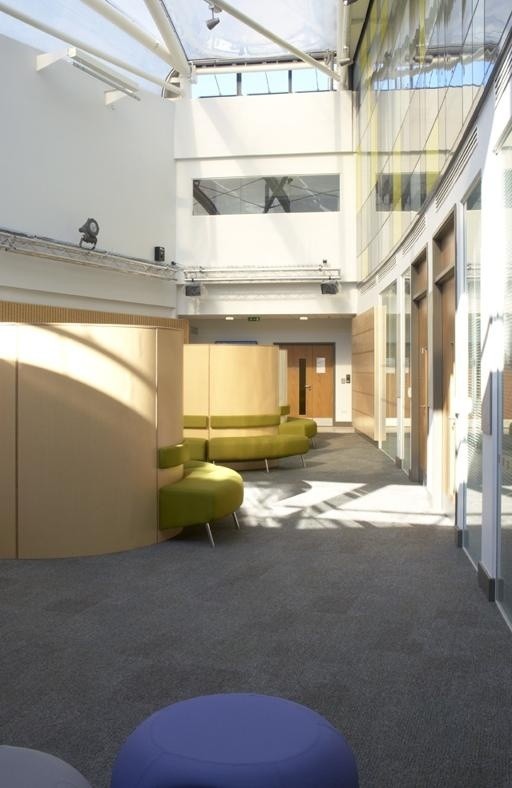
[210,434,310,472]
[159,459,245,547]
[279,416,318,449]
[183,437,207,461]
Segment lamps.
[185,283,201,297]
[78,217,100,250]
[320,280,338,295]
[206,8,220,30]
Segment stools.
[0,744,91,788]
[113,695,359,788]
